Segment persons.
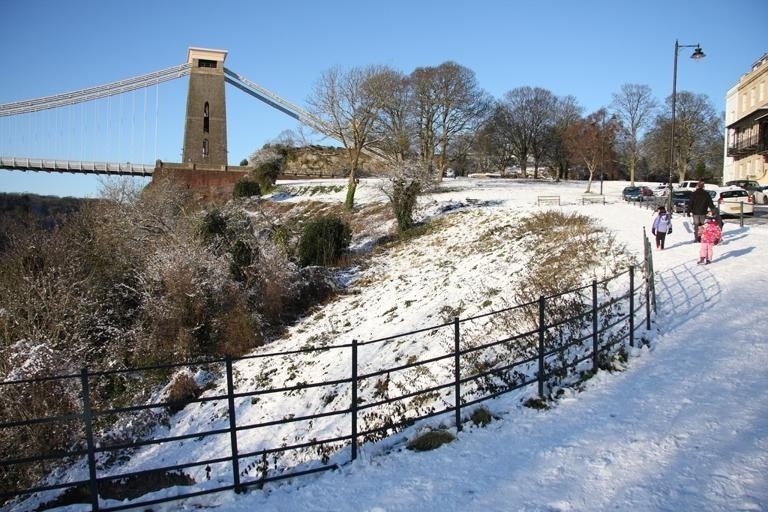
[697,213,721,264]
[687,181,717,241]
[652,206,674,249]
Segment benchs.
[581,194,605,205]
[538,196,560,206]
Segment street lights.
[669,39,707,182]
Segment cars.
[651,180,768,218]
[623,186,656,202]
